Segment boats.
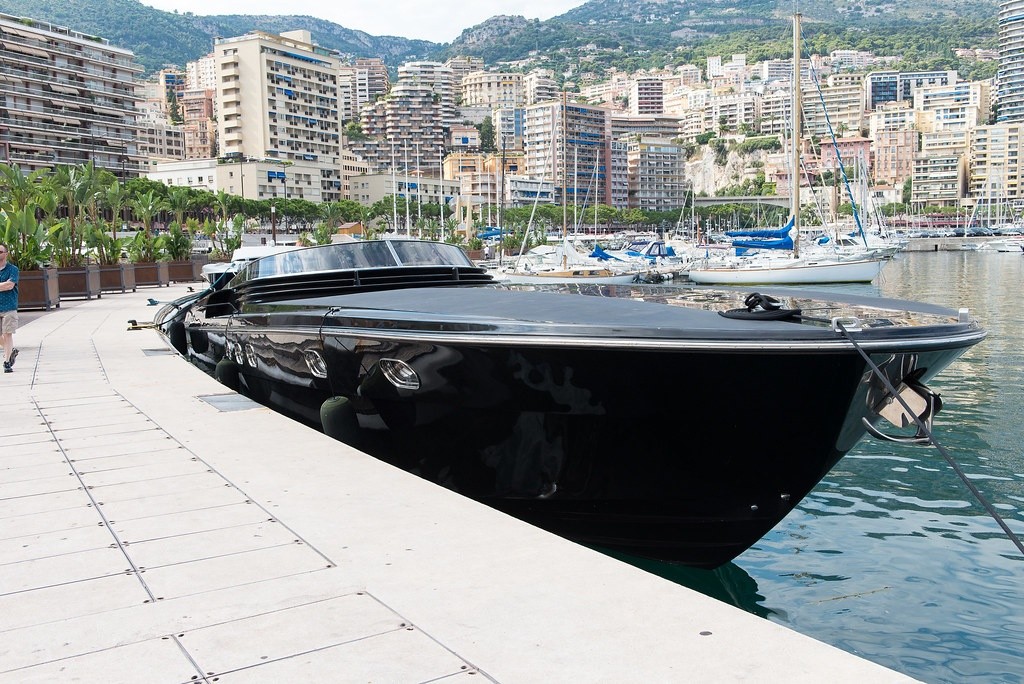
[170,239,991,572]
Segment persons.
[0,242,19,373]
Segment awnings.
[404,183,417,188]
[268,171,286,178]
[304,156,313,160]
[277,87,294,95]
[309,120,317,124]
[445,197,454,204]
[283,51,320,63]
[276,75,292,82]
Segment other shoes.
[4,361,13,373]
[10,348,19,366]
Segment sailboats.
[198,10,1024,289]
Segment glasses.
[0,251,7,255]
